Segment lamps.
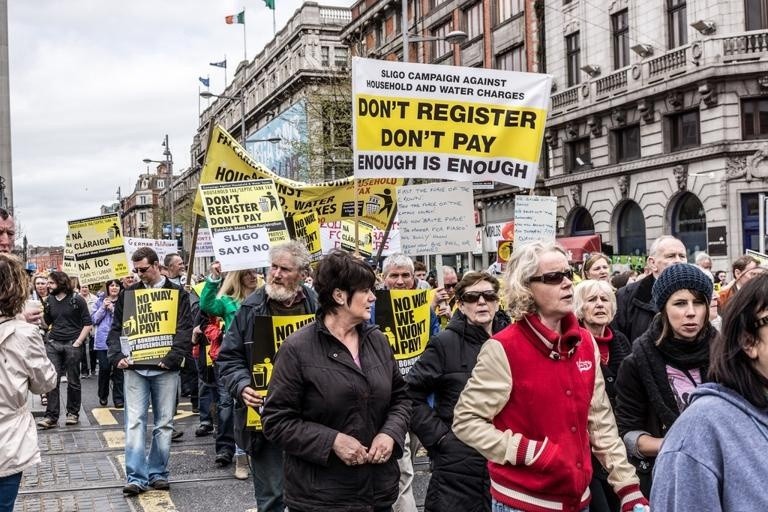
[630,42,654,58]
[578,62,602,77]
[689,19,717,37]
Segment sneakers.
[172,398,216,440]
[61,370,97,384]
[36,392,79,428]
[121,480,170,494]
[99,398,124,409]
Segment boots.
[216,445,249,480]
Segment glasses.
[132,264,152,273]
[444,282,457,290]
[528,269,574,286]
[460,290,497,304]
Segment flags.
[260,0,274,11]
[224,10,245,25]
[207,59,226,69]
[198,76,209,88]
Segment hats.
[611,270,632,289]
[651,262,715,312]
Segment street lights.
[139,152,196,241]
[400,30,473,60]
[191,89,285,160]
